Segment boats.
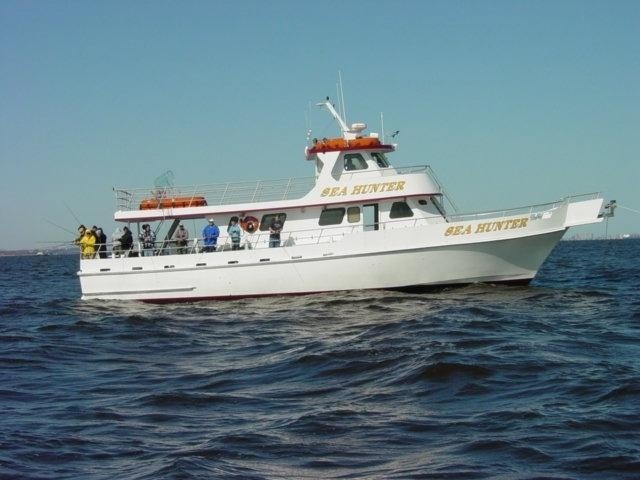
[76,67,613,301]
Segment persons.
[228,218,241,251]
[175,223,188,254]
[112,227,123,257]
[267,214,284,249]
[139,224,145,256]
[115,226,133,259]
[71,224,107,258]
[198,217,220,253]
[138,224,156,257]
